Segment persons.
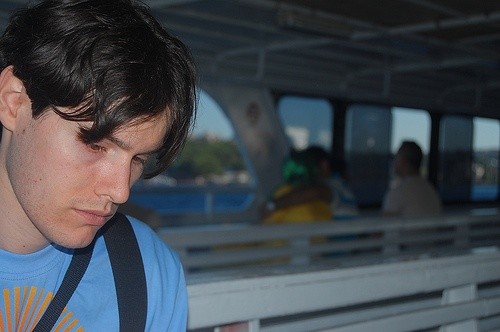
[264,151,333,267]
[357,140,441,252]
[259,144,356,256]
[0,1,198,332]
